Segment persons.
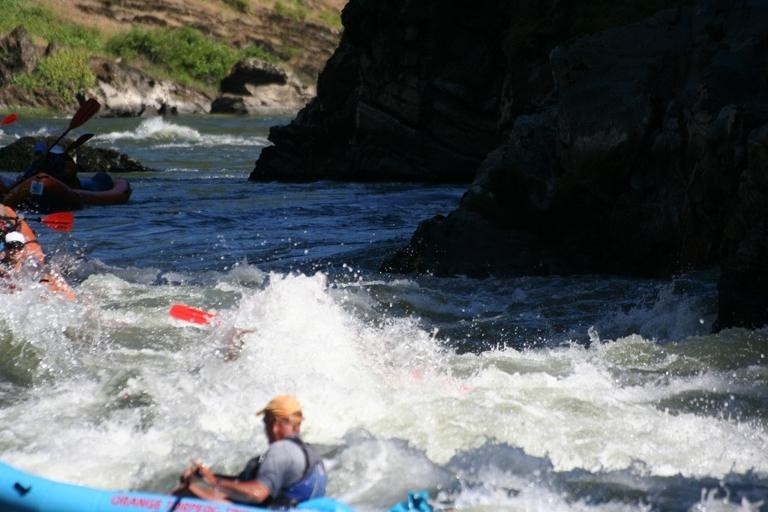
[172,393,327,508]
[16,140,81,190]
[0,204,40,290]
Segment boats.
[1,174,133,213]
[0,457,432,512]
[0,203,80,304]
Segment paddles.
[0,212,74,232]
[0,113,17,125]
[168,304,225,329]
[62,133,95,156]
[47,97,101,151]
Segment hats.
[255,393,306,424]
[4,230,26,246]
